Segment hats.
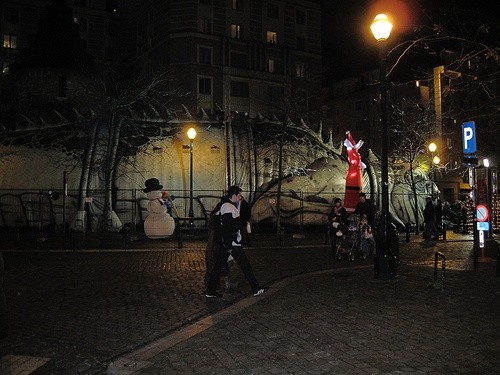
[334,198,342,203]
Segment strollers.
[334,214,369,262]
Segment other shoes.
[253,287,269,297]
[206,292,223,297]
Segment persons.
[353,192,378,254]
[204,186,266,299]
[143,178,175,238]
[328,198,347,255]
[423,197,446,241]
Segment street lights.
[369,10,393,283]
[483,158,493,239]
[428,142,440,187]
[187,126,197,228]
